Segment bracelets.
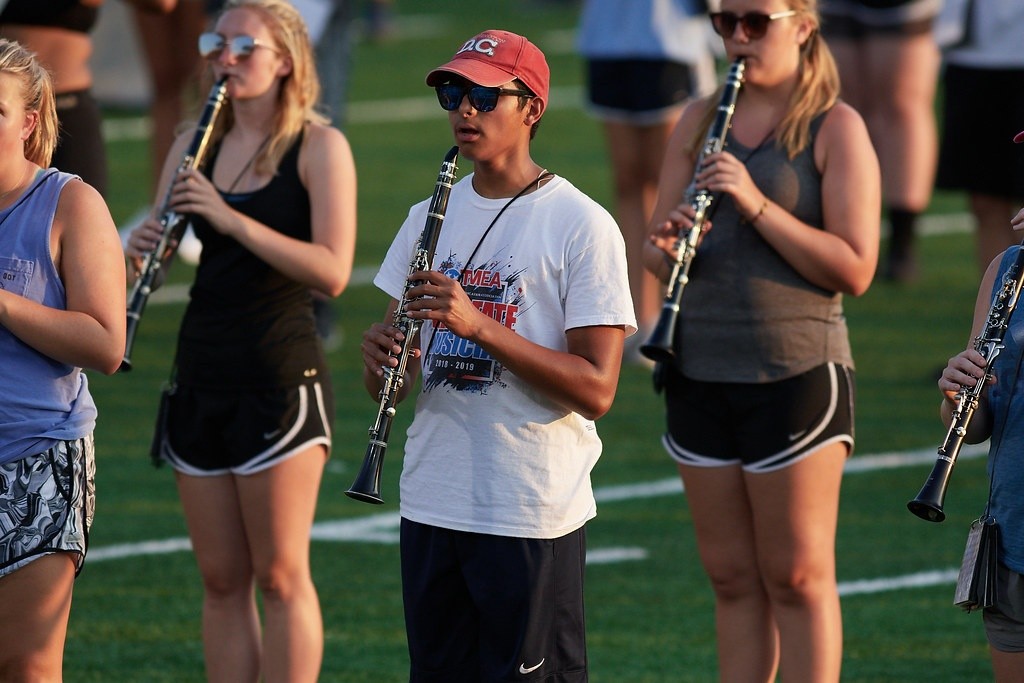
[746,198,768,223]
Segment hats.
[425,30,550,110]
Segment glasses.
[709,10,798,40]
[434,81,533,114]
[197,31,282,64]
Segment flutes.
[345,145,464,505]
[640,57,745,361]
[908,251,1024,524]
[121,72,229,370]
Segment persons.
[0,0,381,356]
[642,0,884,683]
[937,128,1024,683]
[0,38,125,683]
[360,29,639,683]
[124,0,358,682]
[577,0,1024,375]
